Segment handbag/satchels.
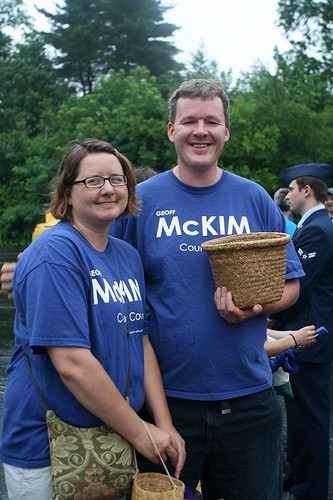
[47,394,139,500]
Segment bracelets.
[289,333,302,351]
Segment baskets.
[200,232,291,309]
[131,418,185,500]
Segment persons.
[2,78,305,500]
[274,187,298,241]
[322,188,332,219]
[282,162,333,499]
[0,138,188,500]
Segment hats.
[326,186,333,195]
[284,163,332,188]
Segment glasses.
[70,175,127,188]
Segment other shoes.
[281,491,296,500]
[282,470,294,492]
